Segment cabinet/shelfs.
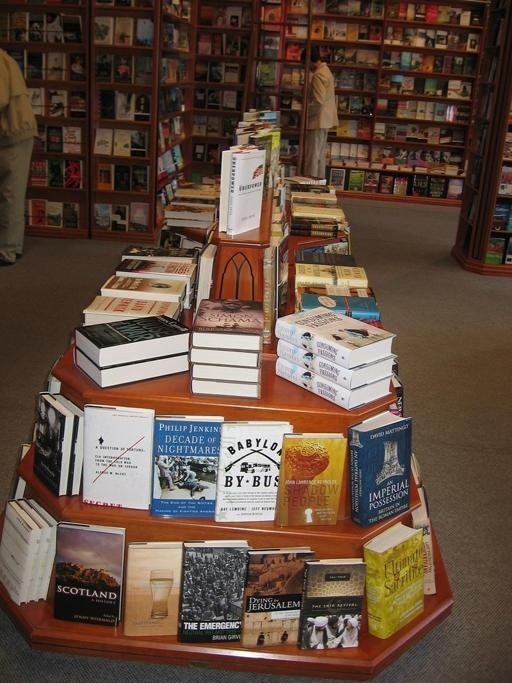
[88,0,199,249]
[305,1,489,215]
[185,2,261,182]
[0,0,97,243]
[252,1,311,177]
[449,9,512,278]
[1,108,456,679]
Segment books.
[437,0,511,265]
